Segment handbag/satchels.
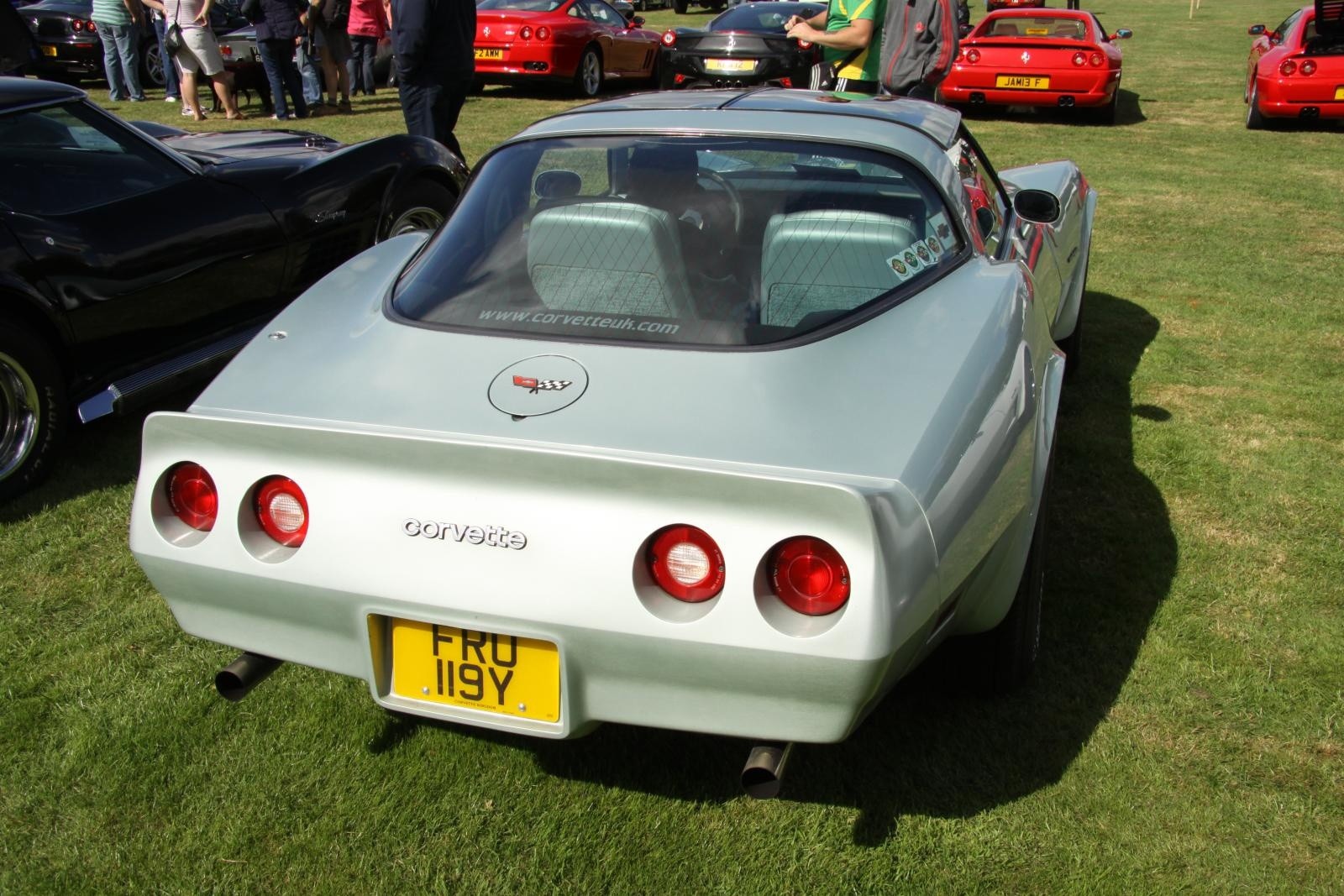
[164,23,181,58]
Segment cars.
[653,1,827,89]
[983,1,1045,12]
[0,1,394,87]
[938,7,1133,127]
[1244,0,1344,132]
[474,0,666,100]
[1,72,474,511]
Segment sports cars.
[126,89,1099,801]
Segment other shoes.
[181,106,207,115]
[289,113,297,118]
[270,114,278,119]
[312,105,338,117]
[337,100,351,113]
[165,96,176,102]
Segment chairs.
[1054,24,1080,37]
[519,199,698,321]
[760,212,922,326]
[994,23,1017,35]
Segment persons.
[295,0,391,115]
[878,0,959,101]
[141,0,248,121]
[91,0,146,101]
[784,0,888,93]
[150,9,180,102]
[389,0,477,163]
[1067,0,1080,11]
[240,0,310,120]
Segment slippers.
[227,114,249,120]
[201,114,207,119]
